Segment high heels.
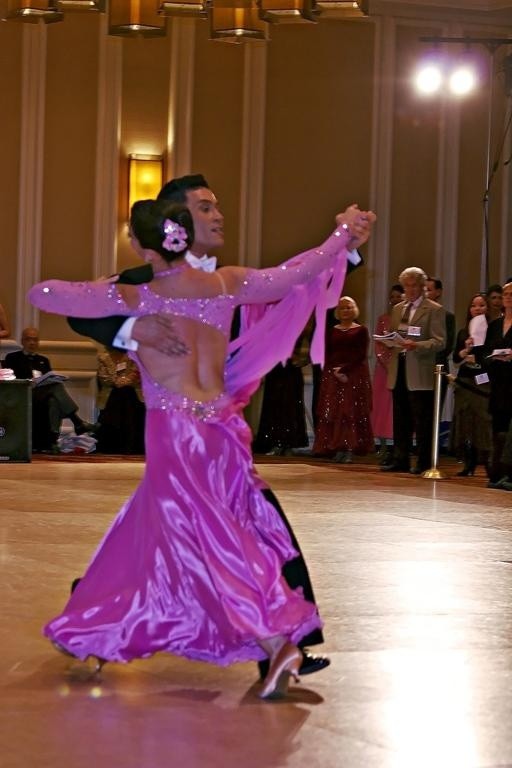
[255,641,305,700]
[50,638,110,672]
[456,458,477,476]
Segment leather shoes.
[382,459,411,473]
[258,649,331,681]
[409,460,432,473]
[398,303,413,338]
[74,421,101,436]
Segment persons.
[95,345,145,454]
[67,174,378,683]
[250,270,510,493]
[5,328,100,451]
[29,199,366,700]
[0,304,11,338]
